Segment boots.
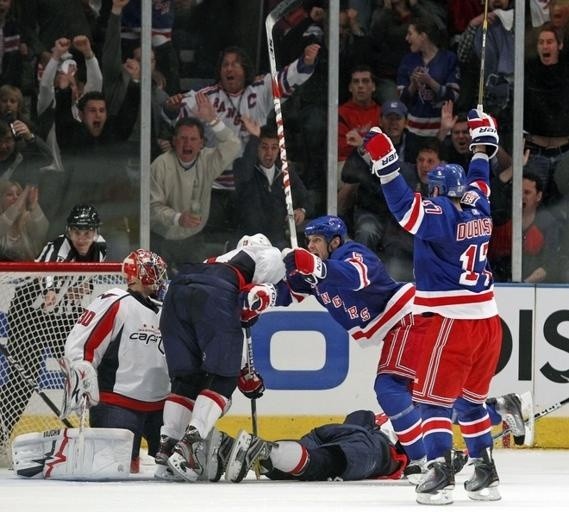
[154,434,179,466]
[174,424,205,476]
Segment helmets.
[424,162,468,199]
[304,214,349,250]
[66,200,103,230]
[121,248,169,287]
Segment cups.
[190,200,203,214]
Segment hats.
[380,98,409,120]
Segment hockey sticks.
[244,325,262,479]
[266,1,299,251]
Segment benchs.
[177,46,226,94]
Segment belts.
[524,140,569,159]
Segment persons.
[362,106,505,506]
[289,214,535,449]
[153,231,289,483]
[0,205,109,446]
[223,408,409,483]
[0,1,568,286]
[64,246,174,474]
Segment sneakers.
[463,459,499,493]
[493,392,526,446]
[403,447,468,496]
[228,429,279,483]
[206,428,237,482]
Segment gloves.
[236,279,279,329]
[237,363,265,399]
[69,360,101,410]
[467,107,500,160]
[357,126,401,178]
[281,246,328,286]
